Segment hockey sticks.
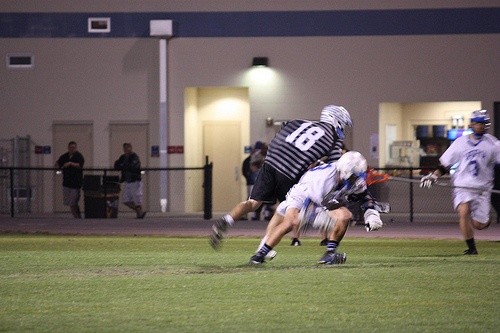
[365,168,500,193]
[296,198,371,238]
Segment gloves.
[363,208,383,232]
[420,172,438,190]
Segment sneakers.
[250,255,265,264]
[319,253,345,264]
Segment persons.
[209,104,353,260]
[420,110,500,255]
[56,141,84,219]
[114,142,147,219]
[250,150,384,265]
[241,141,273,221]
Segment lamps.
[252,57,269,68]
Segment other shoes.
[209,217,228,249]
[268,249,276,259]
[320,238,329,245]
[252,217,260,220]
[265,216,272,221]
[292,239,300,246]
[138,211,147,219]
[464,248,477,255]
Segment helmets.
[320,105,352,139]
[337,150,367,182]
[470,109,491,124]
